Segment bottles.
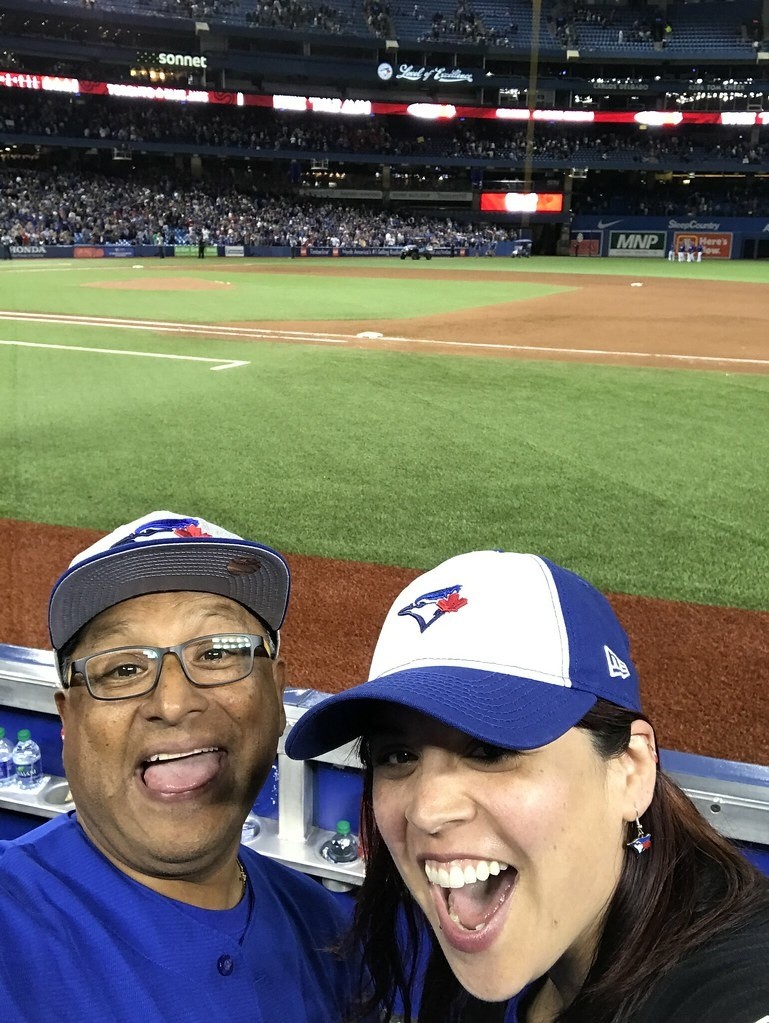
[327,820,358,863]
[0,727,15,789]
[12,729,42,789]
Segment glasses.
[68,633,275,701]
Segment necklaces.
[236,859,246,889]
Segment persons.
[283,550,768,1023]
[668,243,703,262]
[1,0,769,250]
[1,510,378,1023]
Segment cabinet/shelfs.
[1,643,769,896]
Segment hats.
[49,511,292,693]
[284,550,641,762]
